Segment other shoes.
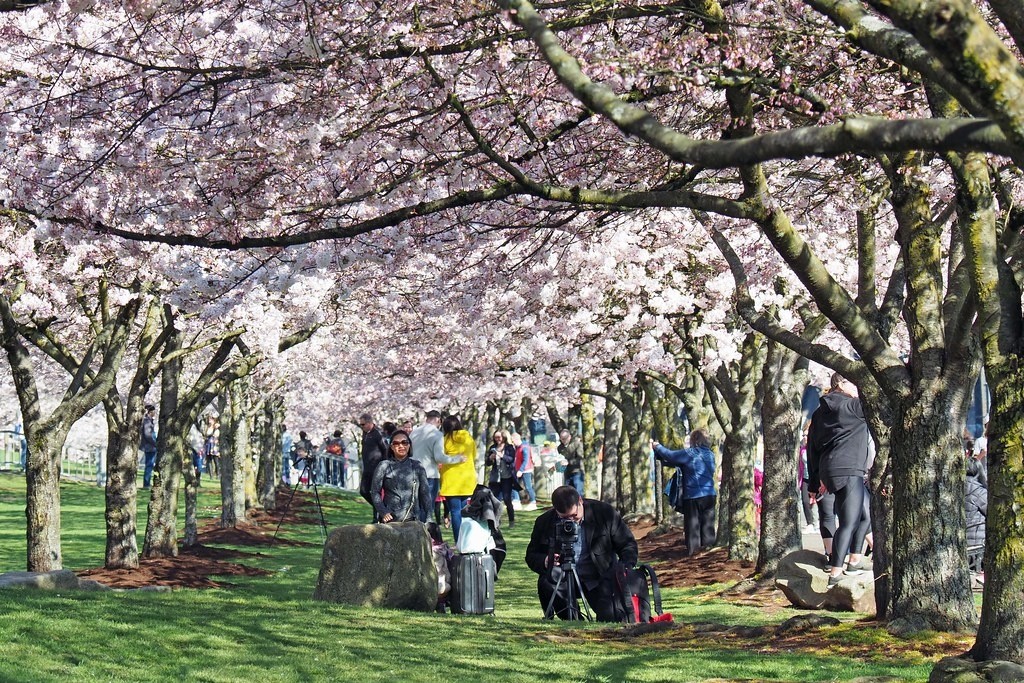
[512,500,524,513]
[800,524,824,535]
[975,569,985,587]
[432,600,451,614]
[509,519,515,531]
[525,501,538,511]
[143,483,154,490]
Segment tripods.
[545,542,593,622]
[269,461,329,548]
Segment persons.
[434,485,450,529]
[189,417,347,495]
[548,429,585,498]
[15,422,26,473]
[371,430,431,523]
[439,415,476,544]
[510,431,539,511]
[403,421,413,436]
[800,372,989,589]
[486,429,524,529]
[360,414,388,523]
[380,422,397,444]
[541,440,566,497]
[409,410,467,523]
[649,427,717,559]
[139,405,157,490]
[524,486,639,623]
[425,522,454,614]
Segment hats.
[334,430,343,438]
[300,430,307,436]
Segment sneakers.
[825,571,853,589]
[843,555,873,576]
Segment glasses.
[555,504,579,519]
[359,422,367,426]
[388,440,410,448]
[493,434,503,440]
[559,436,570,441]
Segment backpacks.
[595,560,675,626]
[663,467,684,514]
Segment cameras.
[304,455,317,463]
[557,520,579,543]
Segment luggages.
[455,552,497,615]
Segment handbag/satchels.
[528,445,541,468]
[456,516,491,556]
[556,457,569,472]
